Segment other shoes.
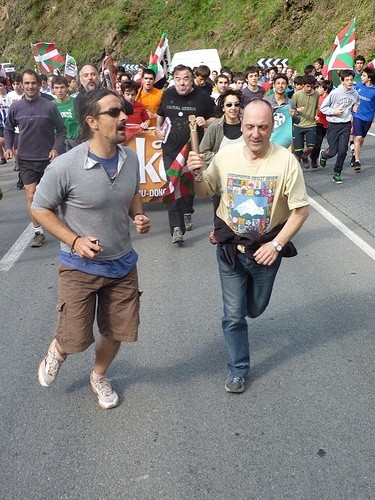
[17,172,24,190]
[350,144,354,152]
[301,156,310,169]
[0,158,7,165]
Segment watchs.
[270,240,282,252]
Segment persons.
[0,57,375,408]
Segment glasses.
[94,107,126,118]
[226,103,240,107]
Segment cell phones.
[90,239,99,253]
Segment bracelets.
[71,236,81,254]
[133,213,147,220]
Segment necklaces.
[89,147,95,155]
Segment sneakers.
[90,370,119,409]
[225,373,245,393]
[37,338,68,387]
[309,153,318,168]
[30,231,45,246]
[332,172,342,184]
[184,213,192,231]
[319,149,326,168]
[350,156,361,172]
[172,227,184,243]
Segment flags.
[30,18,375,85]
[162,139,194,207]
